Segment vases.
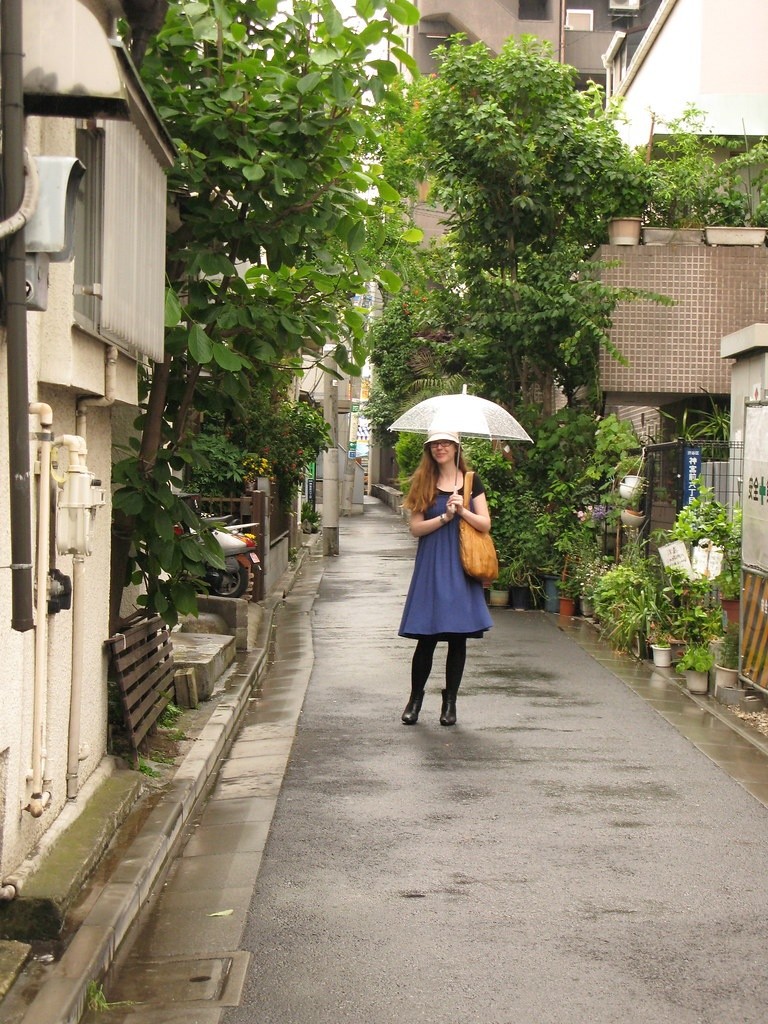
[619,482,644,500]
[578,595,594,617]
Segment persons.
[397,431,494,727]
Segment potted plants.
[675,638,713,695]
[606,143,644,246]
[544,554,562,612]
[489,567,509,605]
[641,100,705,246]
[672,473,742,631]
[650,632,672,667]
[705,161,768,247]
[553,577,576,616]
[664,564,718,664]
[714,618,739,697]
[620,503,646,528]
[618,455,645,487]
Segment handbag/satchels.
[459,471,498,581]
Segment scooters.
[181,516,262,597]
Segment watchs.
[440,513,446,525]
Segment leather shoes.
[440,689,457,725]
[402,690,425,723]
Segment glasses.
[430,441,454,448]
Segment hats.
[424,431,464,451]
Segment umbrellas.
[388,383,534,514]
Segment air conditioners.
[607,0,639,12]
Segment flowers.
[576,556,615,600]
